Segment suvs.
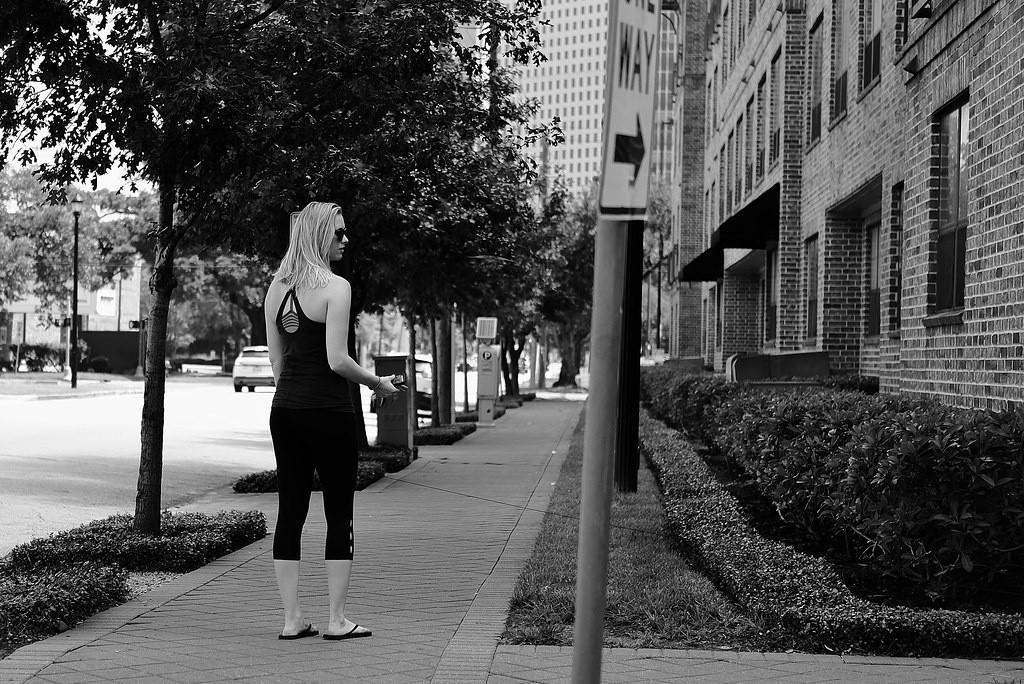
[370,349,433,413]
[232,345,276,392]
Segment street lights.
[71,191,84,388]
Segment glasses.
[335,228,348,241]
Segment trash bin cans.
[371,354,418,469]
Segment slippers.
[323,624,372,640]
[279,623,319,639]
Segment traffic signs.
[597,0,663,223]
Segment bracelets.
[368,375,381,390]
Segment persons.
[264,202,400,640]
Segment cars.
[458,353,528,374]
